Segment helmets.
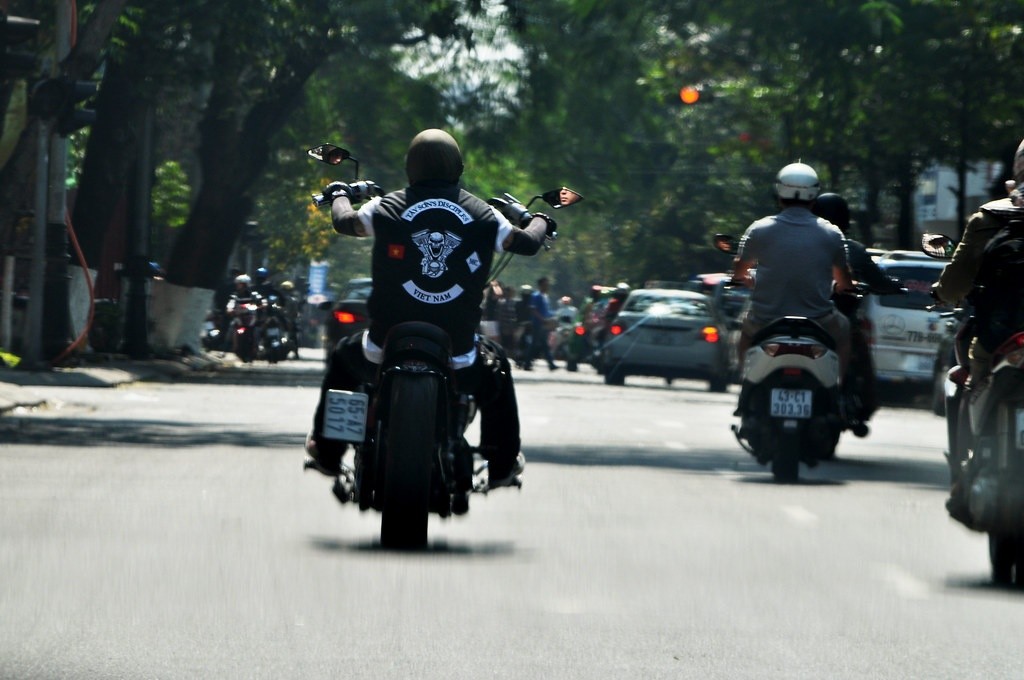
[813,193,850,220]
[616,283,630,295]
[406,129,463,185]
[1013,139,1024,181]
[776,163,820,200]
[234,275,252,289]
[281,281,294,291]
[256,268,268,277]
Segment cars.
[602,289,743,393]
[714,278,755,324]
[855,246,954,384]
[316,276,375,363]
[694,273,733,296]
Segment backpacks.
[971,220,1024,362]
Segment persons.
[930,140,1023,494]
[814,191,891,408]
[227,266,720,373]
[306,128,556,489]
[734,162,865,422]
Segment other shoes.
[306,441,339,474]
[488,451,525,486]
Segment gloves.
[529,212,557,237]
[323,181,353,205]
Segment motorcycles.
[690,234,910,484]
[226,293,290,364]
[919,229,1023,589]
[307,142,584,550]
